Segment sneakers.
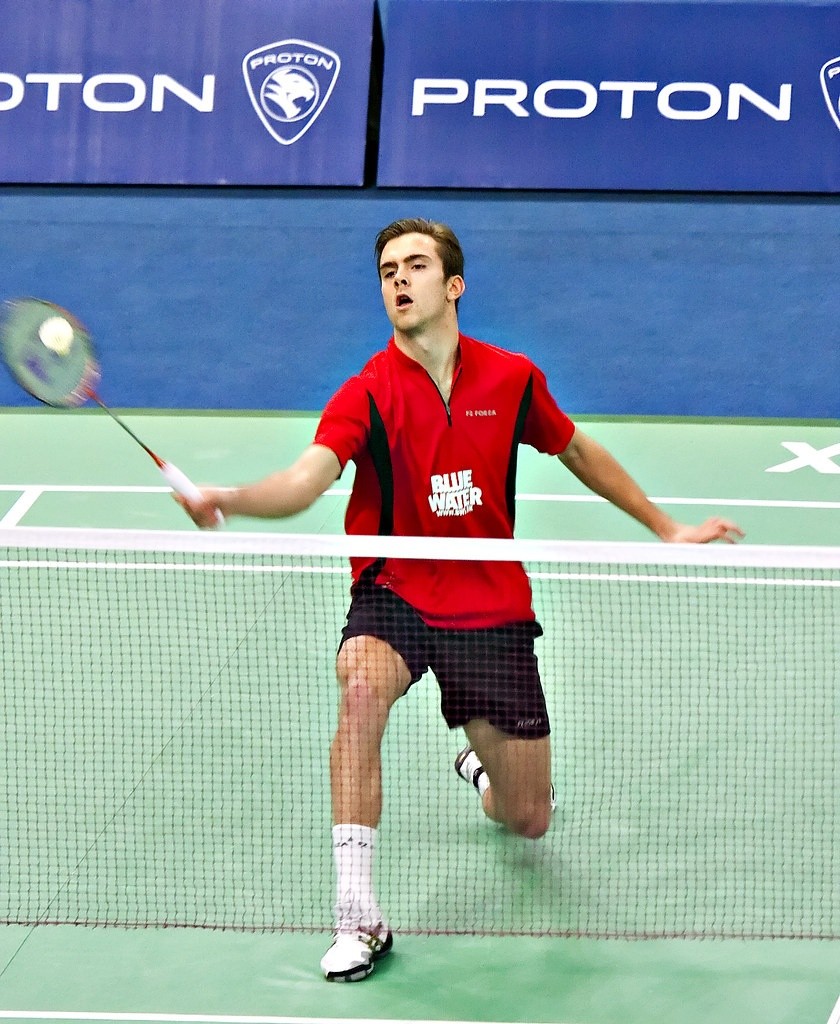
[320,924,393,982]
[454,744,556,812]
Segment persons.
[168,215,744,984]
